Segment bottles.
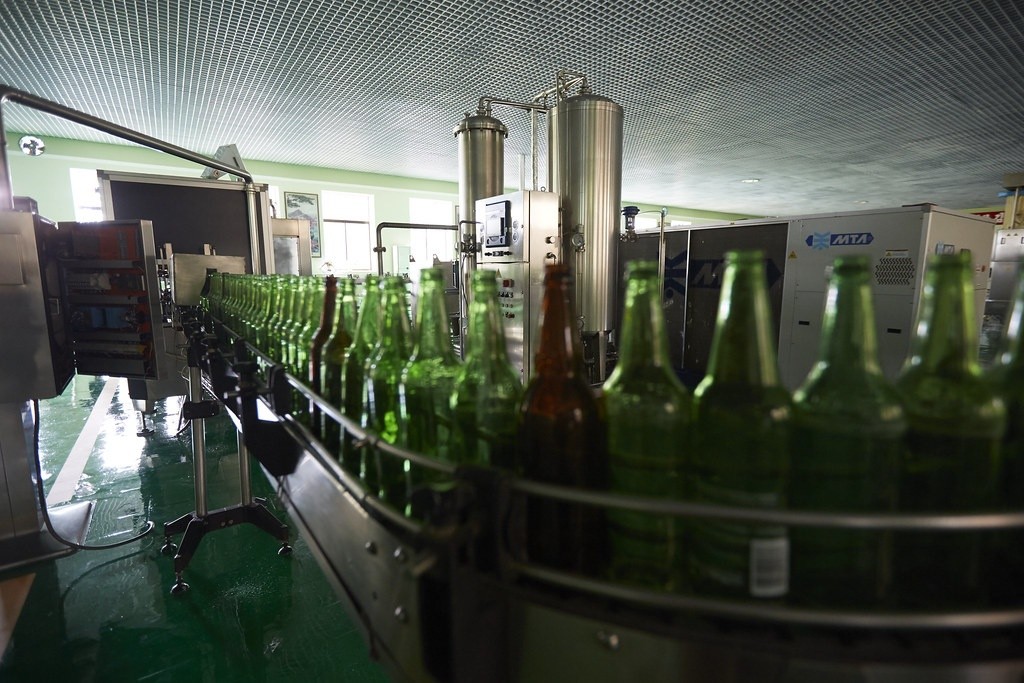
[788,256,912,622]
[974,266,1023,612]
[680,245,799,622]
[888,247,1011,635]
[195,255,689,610]
